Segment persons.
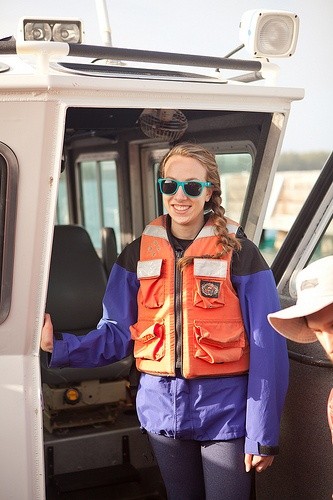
[41,143,288,500]
[267,256,332,437]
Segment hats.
[267,254,333,344]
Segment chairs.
[40,224,133,389]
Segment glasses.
[157,178,210,198]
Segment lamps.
[214,7,301,75]
[22,18,83,45]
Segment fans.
[137,108,190,141]
